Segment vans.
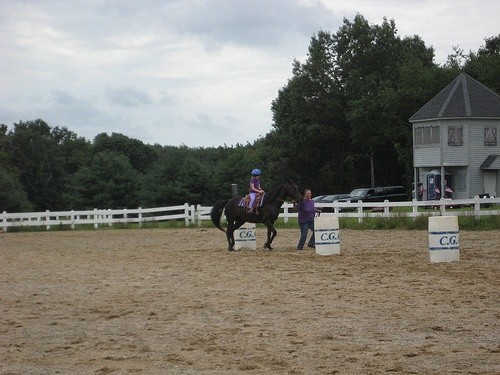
[338,185,408,203]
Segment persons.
[247,168,265,215]
[294,189,320,250]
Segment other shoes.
[298,247,302,250]
[248,208,254,214]
[308,245,315,247]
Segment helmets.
[251,169,261,176]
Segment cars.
[311,194,357,213]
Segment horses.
[209,172,304,252]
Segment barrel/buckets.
[428,216,460,263]
[314,216,342,255]
[233,221,256,251]
